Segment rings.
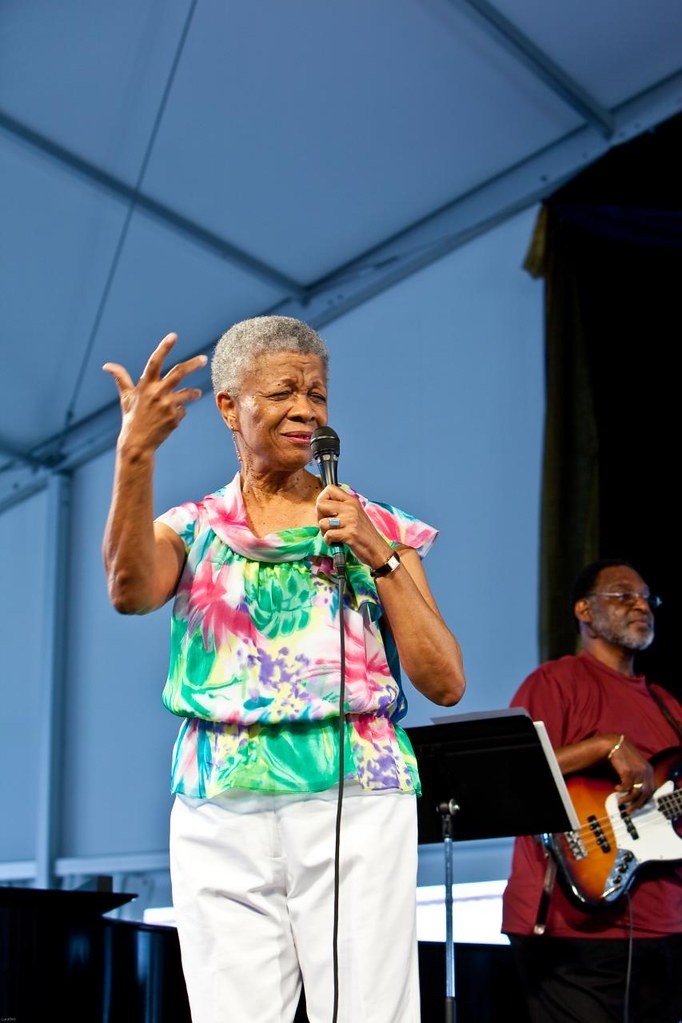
[633,783,644,788]
[328,517,340,529]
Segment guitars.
[539,741,681,917]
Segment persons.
[102,316,466,1023]
[501,558,682,1023]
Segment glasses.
[584,591,662,609]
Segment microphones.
[309,424,348,576]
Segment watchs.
[370,551,401,577]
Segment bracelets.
[608,735,624,759]
